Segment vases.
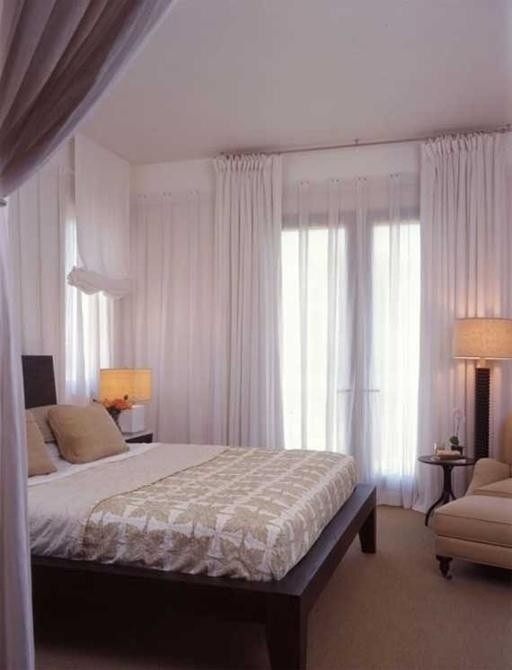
[110,413,123,433]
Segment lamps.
[447,314,512,458]
[98,366,156,406]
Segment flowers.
[96,395,135,415]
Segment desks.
[417,452,475,531]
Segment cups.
[433,440,448,457]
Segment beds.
[23,355,383,668]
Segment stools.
[426,493,512,580]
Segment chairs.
[465,417,512,499]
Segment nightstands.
[119,431,155,444]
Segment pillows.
[46,399,131,465]
[25,410,60,479]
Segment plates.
[437,455,464,461]
